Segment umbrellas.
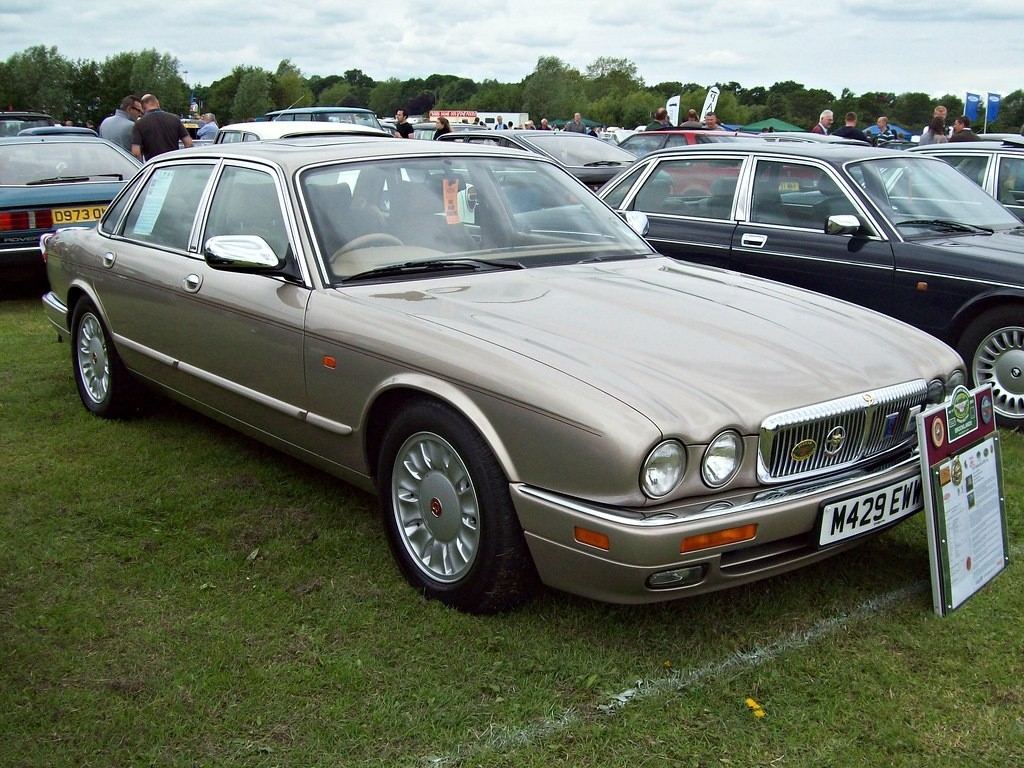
[740,118,807,132]
[536,118,603,131]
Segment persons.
[86,120,99,134]
[395,109,414,139]
[645,108,725,131]
[877,117,895,148]
[462,113,605,140]
[131,94,192,162]
[197,113,219,140]
[811,110,833,136]
[919,106,981,146]
[769,127,774,132]
[99,95,142,152]
[762,128,768,133]
[829,112,873,147]
[433,118,456,141]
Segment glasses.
[131,107,144,117]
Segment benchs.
[213,178,351,245]
[639,176,736,218]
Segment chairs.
[813,173,858,228]
[312,205,380,259]
[979,164,1017,205]
[750,180,794,227]
[390,178,477,252]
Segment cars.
[0,106,1024,197]
[0,135,147,298]
[40,135,973,620]
[503,142,1024,434]
[854,141,1024,225]
